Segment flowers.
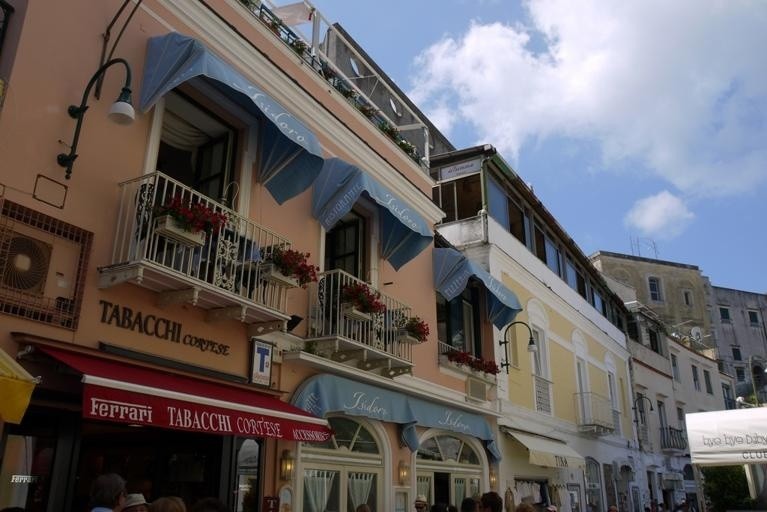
[403,315,430,342]
[441,349,502,376]
[344,282,388,317]
[269,247,320,290]
[152,193,228,237]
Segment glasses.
[415,506,425,510]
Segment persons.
[87,473,128,512]
[187,497,232,511]
[356,504,371,512]
[645,496,712,511]
[413,490,559,512]
[606,505,619,511]
[152,496,188,512]
[120,492,153,512]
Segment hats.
[122,494,152,510]
[546,506,558,512]
[88,473,127,504]
[413,495,429,505]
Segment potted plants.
[443,361,496,382]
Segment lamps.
[280,448,294,481]
[56,58,136,180]
[398,460,409,484]
[499,321,538,375]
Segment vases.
[152,215,206,248]
[397,328,421,345]
[343,302,372,321]
[263,263,301,289]
